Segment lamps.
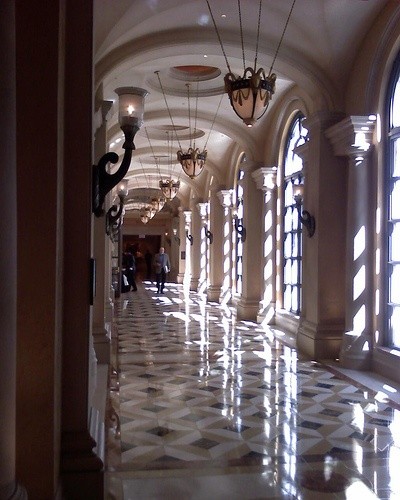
[292,183,316,239]
[206,0,297,128]
[92,67,246,244]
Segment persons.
[124,251,137,292]
[153,247,170,294]
